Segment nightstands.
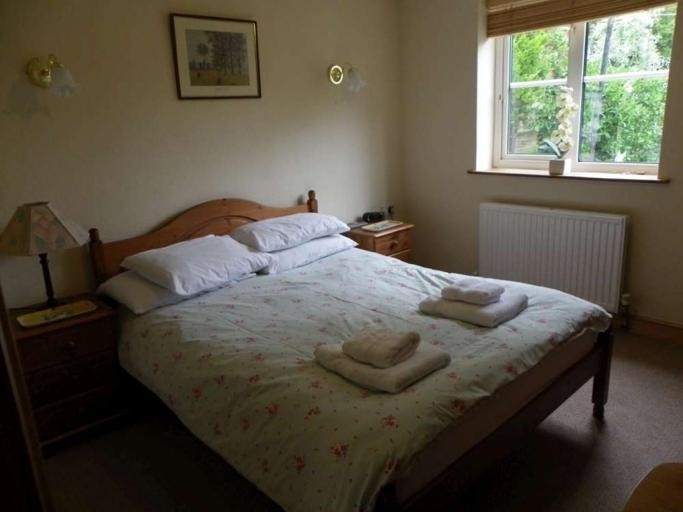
[8,292,130,459]
[350,220,415,263]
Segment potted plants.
[537,139,570,175]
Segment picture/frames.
[169,12,261,100]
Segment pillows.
[100,212,359,315]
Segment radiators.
[477,202,632,315]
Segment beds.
[87,190,615,512]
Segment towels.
[342,324,421,369]
[419,286,528,328]
[441,279,505,305]
[314,341,451,393]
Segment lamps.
[1,201,91,309]
[25,53,65,89]
[327,63,369,92]
